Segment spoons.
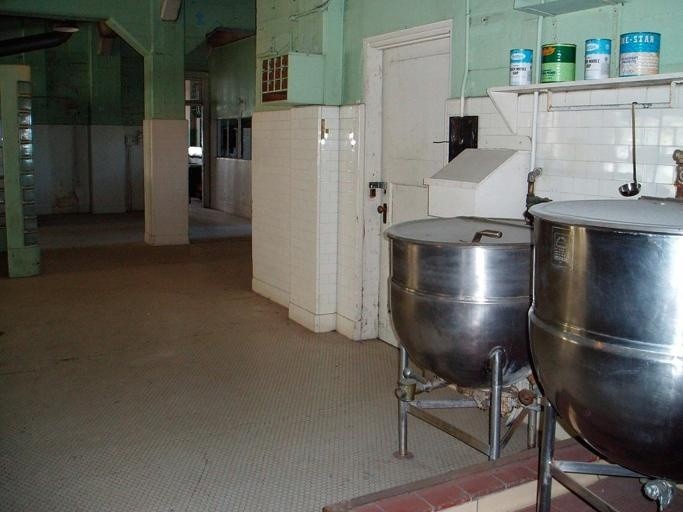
[619,104,642,196]
